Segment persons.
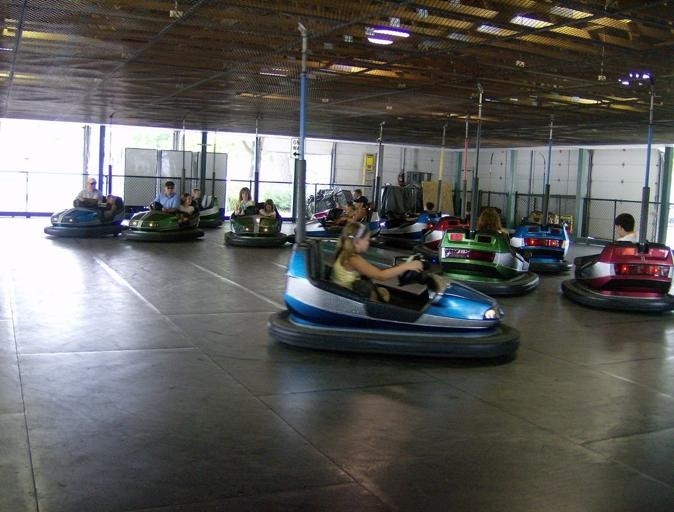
[473,206,509,236]
[325,221,425,305]
[73,177,105,204]
[612,212,639,245]
[109,196,117,212]
[424,201,436,213]
[149,176,370,226]
[103,193,112,212]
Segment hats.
[88,178,96,184]
[165,181,174,186]
[354,196,368,203]
